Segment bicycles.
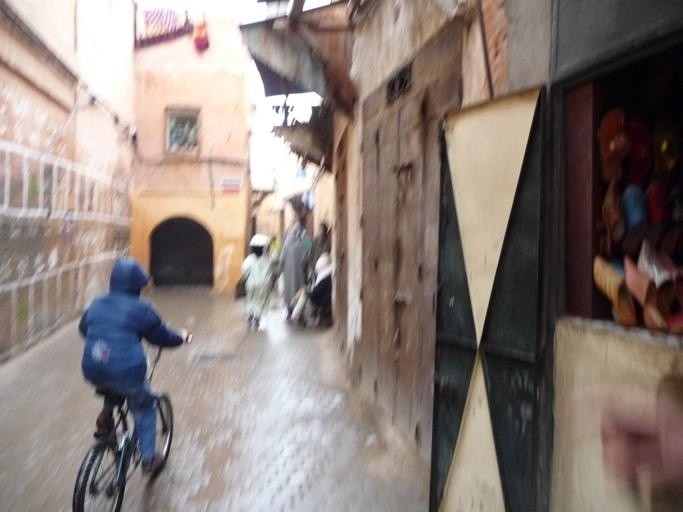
[71,331,193,511]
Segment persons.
[79,258,189,473]
[274,218,331,328]
[240,233,278,331]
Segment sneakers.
[142,452,162,474]
[95,414,114,433]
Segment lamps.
[249,36,323,128]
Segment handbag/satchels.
[236,282,245,297]
